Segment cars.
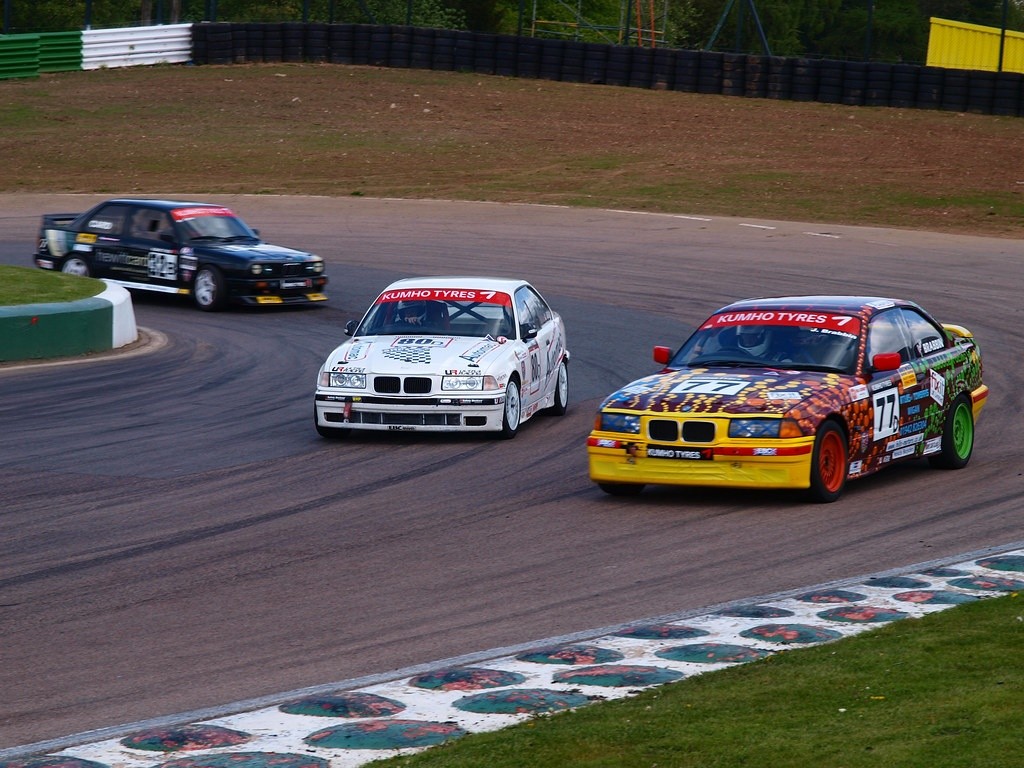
[315,275,570,440]
[586,296,989,503]
[32,198,330,312]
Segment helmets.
[397,299,427,309]
[731,322,767,335]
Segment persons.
[396,299,431,327]
[735,326,794,363]
